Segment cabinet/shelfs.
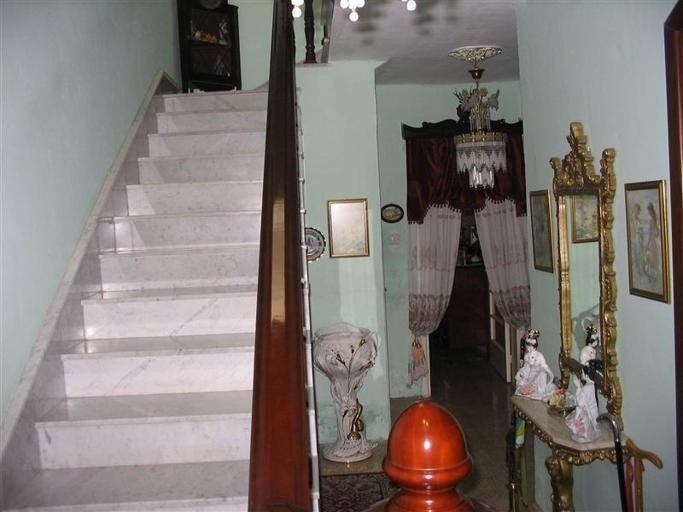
[181,2,244,95]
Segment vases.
[312,320,384,461]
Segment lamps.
[442,45,515,193]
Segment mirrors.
[325,196,372,264]
[550,119,623,431]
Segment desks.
[506,395,631,510]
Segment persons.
[565,324,603,443]
[629,201,659,282]
[512,329,559,403]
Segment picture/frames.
[623,180,670,305]
[528,189,556,274]
[568,192,598,244]
